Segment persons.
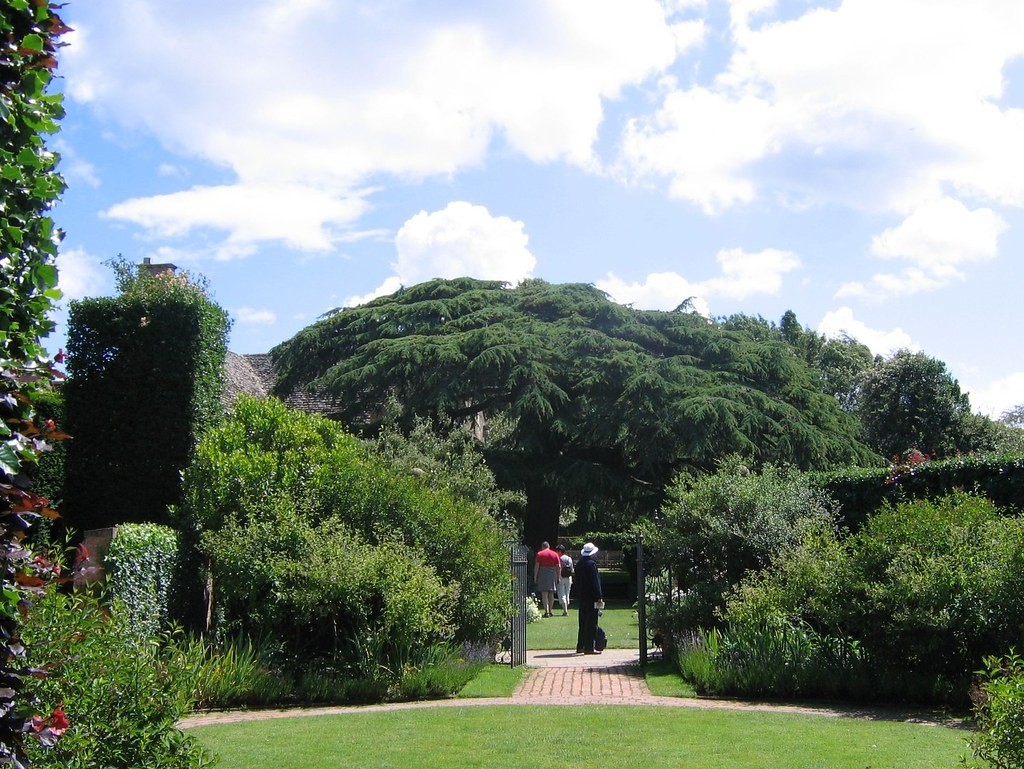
[555,545,573,616]
[534,542,561,618]
[575,542,603,654]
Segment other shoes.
[577,646,601,654]
[542,613,549,618]
[550,613,553,617]
[563,612,567,616]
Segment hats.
[581,543,599,557]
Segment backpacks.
[560,556,571,578]
[594,627,607,651]
